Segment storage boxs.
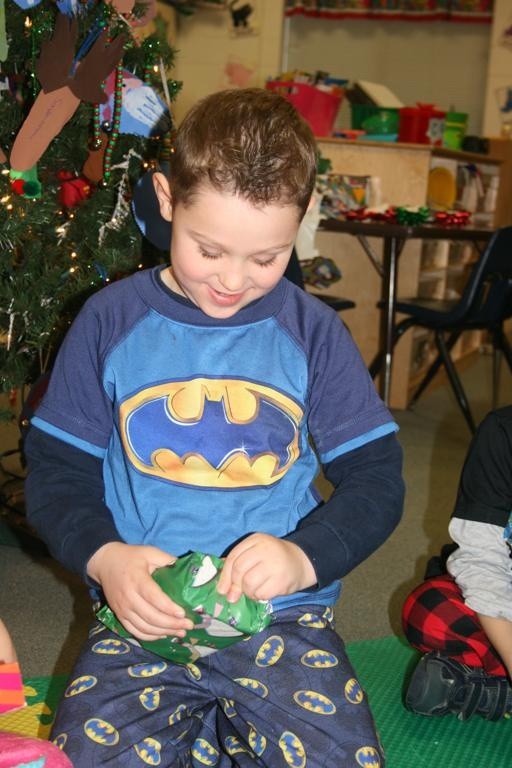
[398,105,447,150]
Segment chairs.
[280,241,356,340]
[369,224,512,437]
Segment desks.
[317,214,511,413]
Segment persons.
[401,405,512,720]
[24,88,405,768]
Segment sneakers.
[401,648,512,719]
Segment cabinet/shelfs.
[309,135,502,411]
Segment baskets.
[264,80,343,139]
